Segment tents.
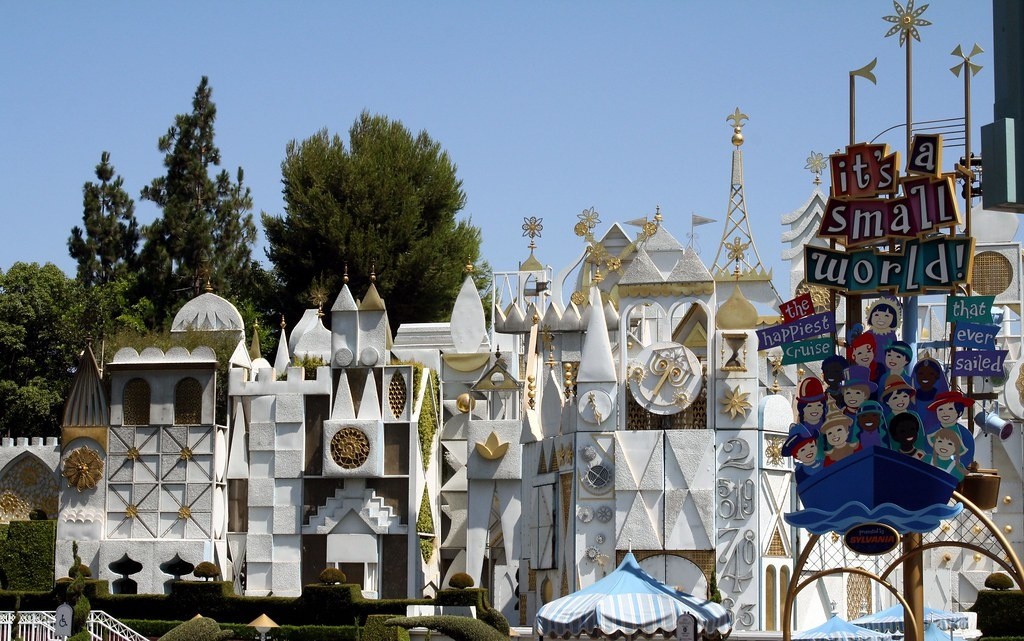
[791,599,892,641]
[848,603,969,641]
[900,612,968,641]
[536,538,732,641]
